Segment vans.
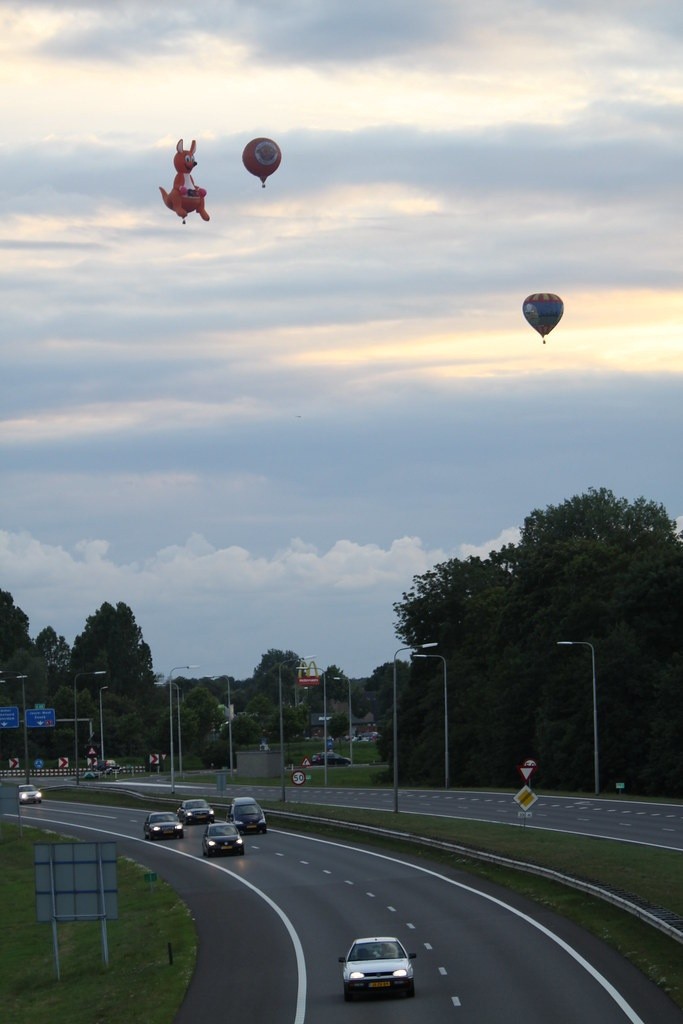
[202,798,266,857]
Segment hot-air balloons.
[243,138,281,187]
[523,293,564,344]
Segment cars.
[102,760,120,771]
[339,937,417,1002]
[143,799,215,840]
[355,732,376,742]
[312,752,351,766]
[16,785,41,805]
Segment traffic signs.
[0,707,55,728]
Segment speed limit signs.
[291,771,305,785]
[523,756,538,775]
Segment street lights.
[297,666,352,785]
[204,676,234,778]
[557,642,599,795]
[74,671,108,786]
[154,665,200,794]
[279,656,318,802]
[394,643,448,812]
[0,670,30,784]
